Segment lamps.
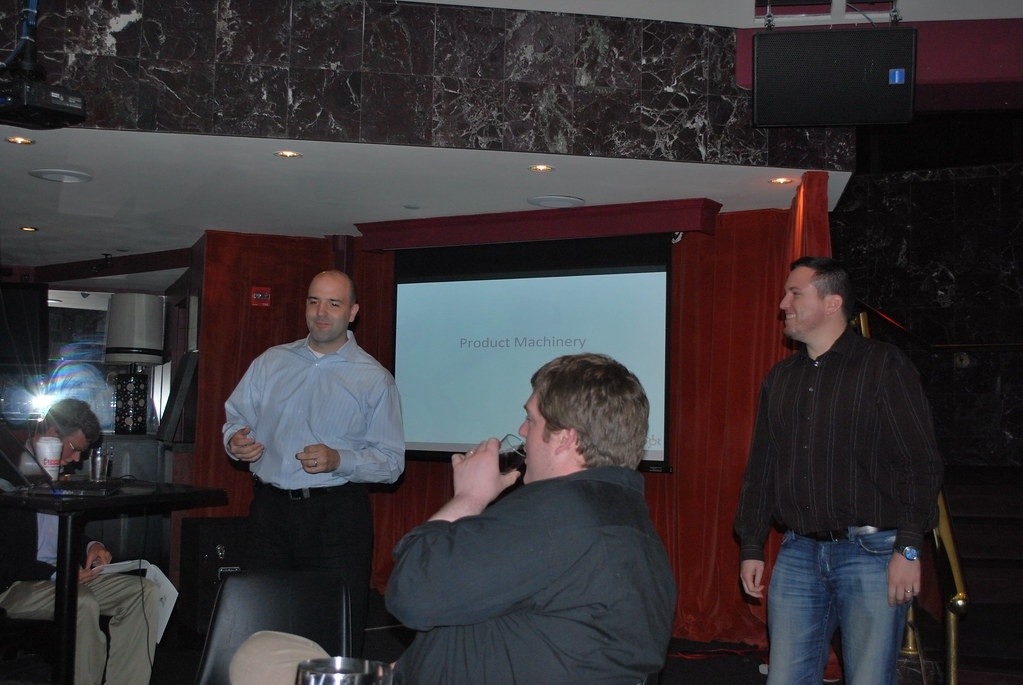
[104,292,165,435]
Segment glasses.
[55,427,88,456]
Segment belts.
[790,525,898,542]
[257,479,368,501]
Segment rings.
[467,451,473,454]
[315,460,317,467]
[905,591,912,592]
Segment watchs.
[893,544,920,560]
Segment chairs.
[191,567,351,684]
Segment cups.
[295,656,394,685]
[498,434,528,476]
[34,437,63,481]
[89,449,107,483]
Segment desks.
[0,477,231,685]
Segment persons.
[0,400,160,685]
[227,353,678,685]
[222,270,404,661]
[732,258,941,685]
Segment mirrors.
[46,267,190,435]
